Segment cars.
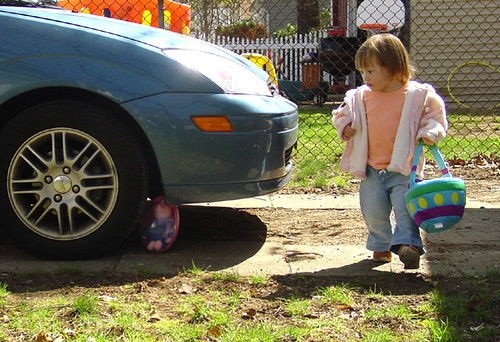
[0,5,299,261]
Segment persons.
[332,33,448,269]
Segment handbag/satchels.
[404,138,466,232]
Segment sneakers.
[373,250,391,262]
[397,244,419,269]
